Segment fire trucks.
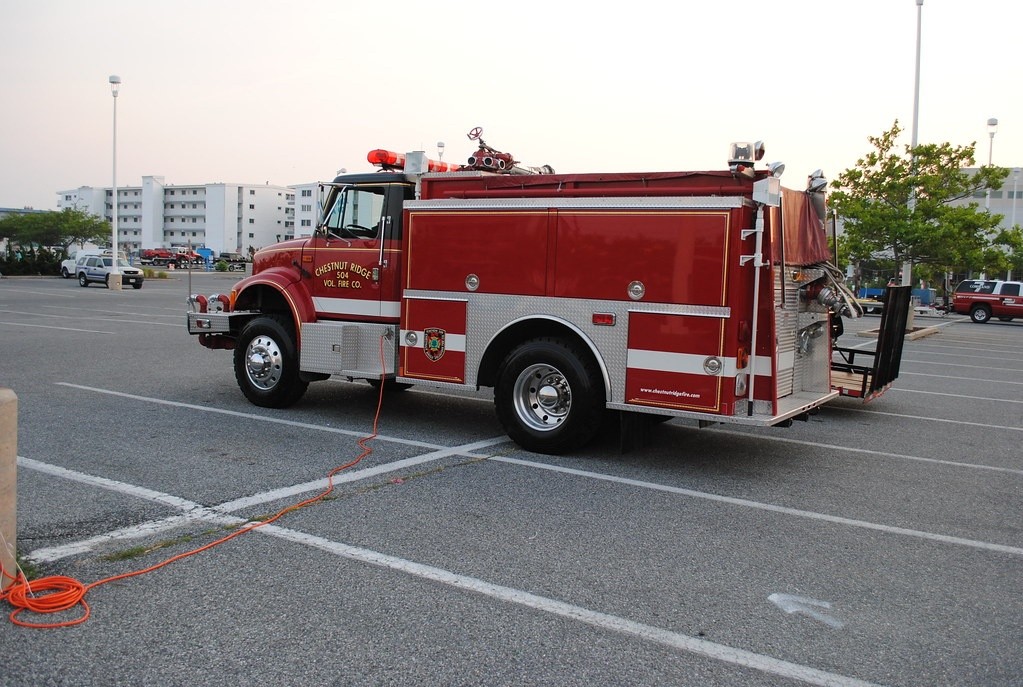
[186,127,914,457]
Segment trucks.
[141,247,204,268]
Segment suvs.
[60,249,109,280]
[74,254,144,288]
[952,279,1022,324]
[212,252,245,272]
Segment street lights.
[108,74,124,292]
[978,117,998,279]
[1005,167,1022,281]
